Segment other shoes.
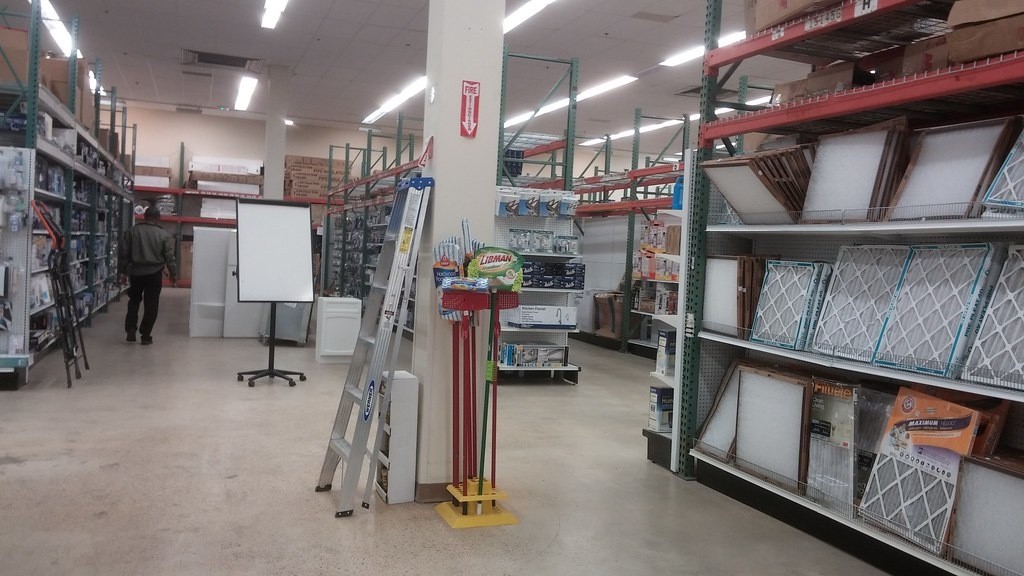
[141,339,153,345]
[127,328,136,342]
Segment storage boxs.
[48,58,94,129]
[163,241,193,288]
[508,305,577,330]
[285,155,352,198]
[0,26,51,90]
[189,170,263,186]
[593,293,624,339]
[134,166,172,179]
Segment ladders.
[314,177,434,520]
[31,199,91,389]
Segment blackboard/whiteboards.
[234,198,319,303]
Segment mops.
[431,218,523,516]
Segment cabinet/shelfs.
[233,197,316,387]
[322,42,691,384]
[678,0,1024,576]
[0,0,264,392]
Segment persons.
[116,205,178,345]
